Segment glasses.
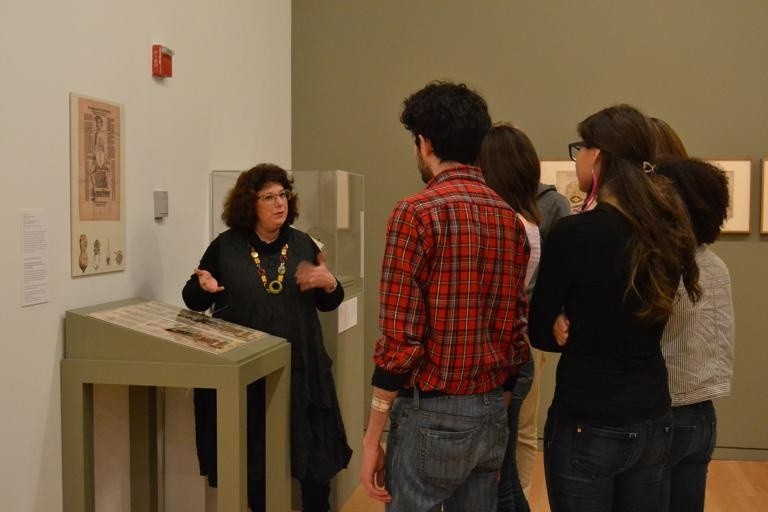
[257,189,292,202]
[567,139,586,161]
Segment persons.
[529,103,701,512]
[182,163,352,511]
[360,78,536,512]
[645,156,735,512]
[517,183,570,499]
[478,121,535,511]
[645,117,690,164]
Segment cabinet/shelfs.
[206,170,365,512]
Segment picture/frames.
[761,157,768,233]
[697,156,752,236]
[69,91,127,278]
[538,156,597,212]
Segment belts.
[394,387,457,398]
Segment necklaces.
[248,244,291,295]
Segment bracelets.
[370,397,392,414]
